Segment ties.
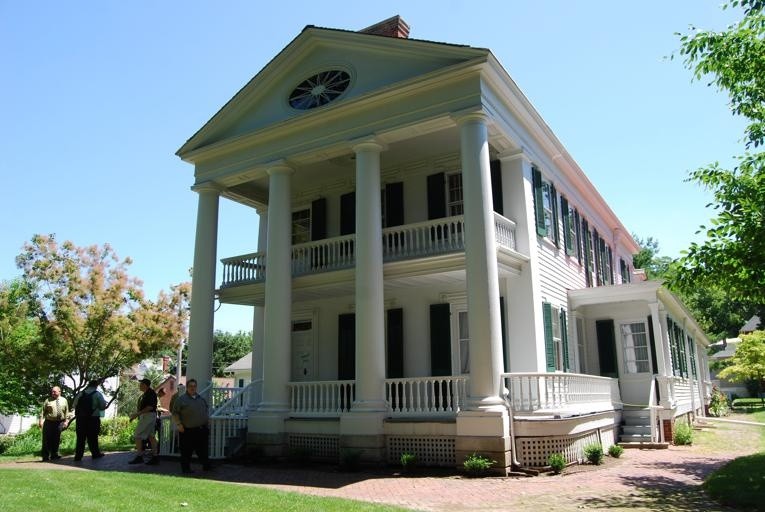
[56,399,62,421]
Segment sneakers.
[74,452,104,461]
[182,465,216,474]
[43,456,61,462]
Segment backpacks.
[75,391,97,419]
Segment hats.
[138,378,151,386]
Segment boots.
[128,455,144,465]
[144,455,161,465]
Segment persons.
[137,384,184,453]
[129,378,161,465]
[39,385,69,460]
[172,380,212,473]
[73,380,107,461]
